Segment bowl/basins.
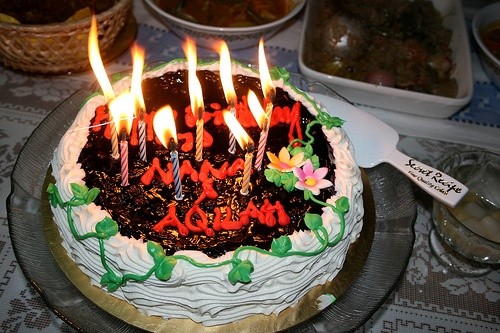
[141,0,308,51]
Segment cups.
[427,154,500,278]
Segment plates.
[299,0,475,120]
[5,61,418,333]
[470,0,500,69]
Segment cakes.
[45,58,364,324]
